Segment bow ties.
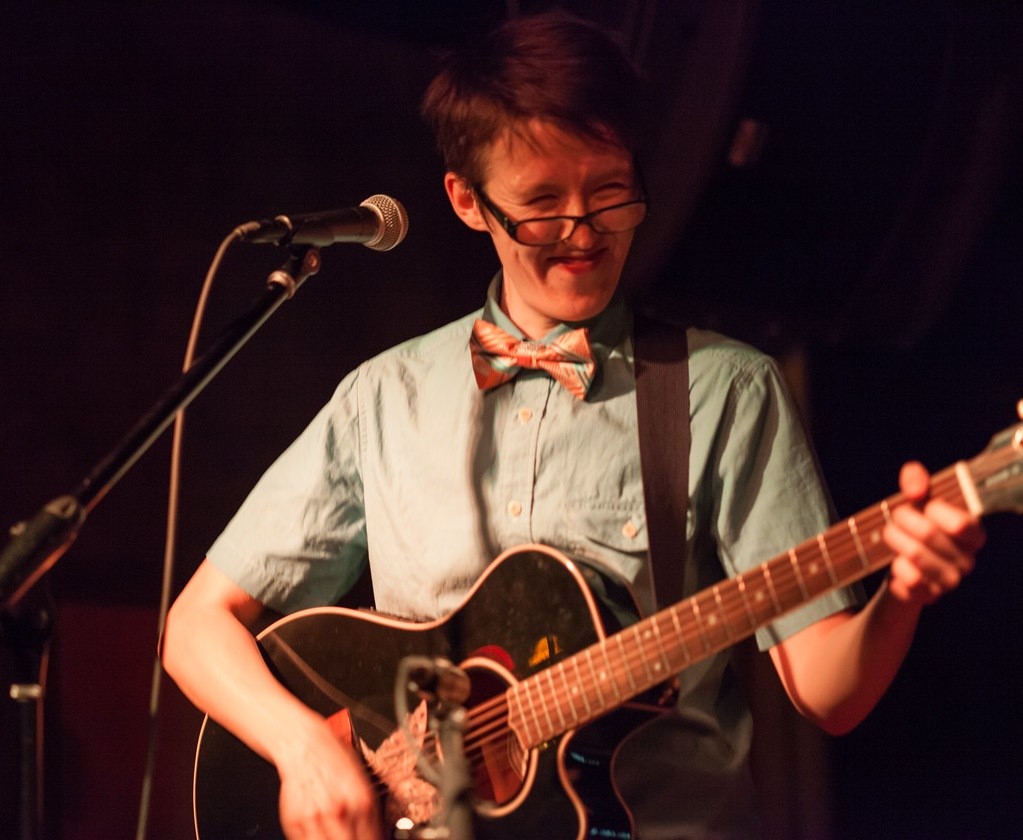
[470,324,597,400]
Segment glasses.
[471,161,652,248]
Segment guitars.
[188,392,1022,839]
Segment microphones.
[229,193,410,252]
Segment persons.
[156,12,986,840]
